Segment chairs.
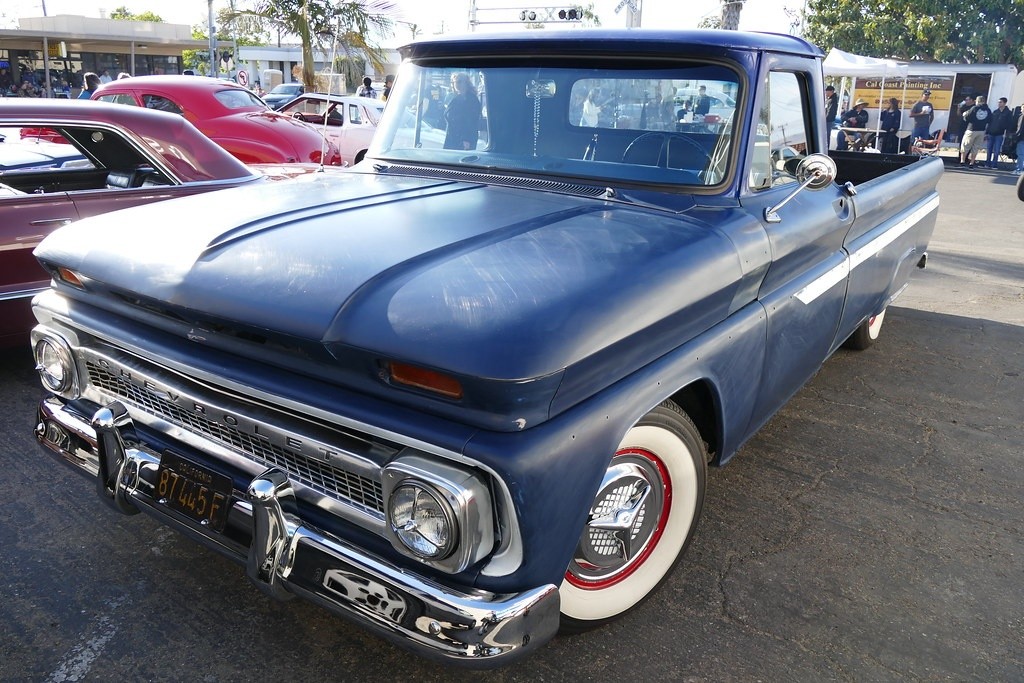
[911,129,945,162]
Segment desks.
[838,128,886,152]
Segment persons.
[380,74,395,102]
[836,98,869,150]
[1010,104,1024,175]
[0,68,12,89]
[954,96,1011,169]
[866,98,901,153]
[358,77,377,99]
[61,70,195,99]
[442,72,482,151]
[908,89,934,148]
[824,86,837,150]
[579,85,711,131]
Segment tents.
[821,46,910,154]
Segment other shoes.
[969,165,975,170]
[978,164,990,169]
[954,163,966,168]
[1010,169,1021,175]
[992,166,998,170]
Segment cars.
[0,98,346,347]
[260,83,304,111]
[273,92,487,167]
[20,75,341,167]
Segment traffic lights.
[519,11,536,20]
[559,9,583,20]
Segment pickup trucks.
[33,29,945,660]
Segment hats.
[853,98,869,109]
[384,75,395,82]
[922,90,931,94]
[825,86,834,91]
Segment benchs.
[106,163,167,190]
[530,129,730,179]
